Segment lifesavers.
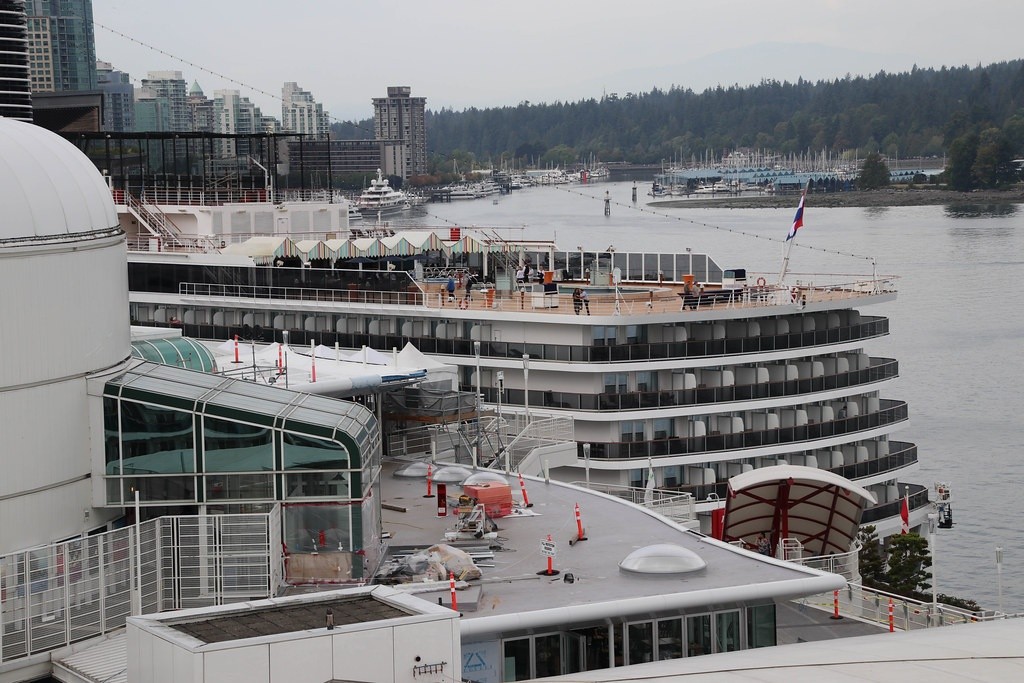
[756,277,766,288]
[459,301,468,310]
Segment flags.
[786,181,809,241]
[644,462,655,506]
[900,495,909,527]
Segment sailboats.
[339,152,611,219]
[652,145,862,193]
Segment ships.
[126,228,934,559]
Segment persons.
[463,274,473,301]
[755,531,772,557]
[445,276,457,300]
[573,288,590,315]
[515,264,545,284]
[837,405,848,418]
[682,279,700,310]
